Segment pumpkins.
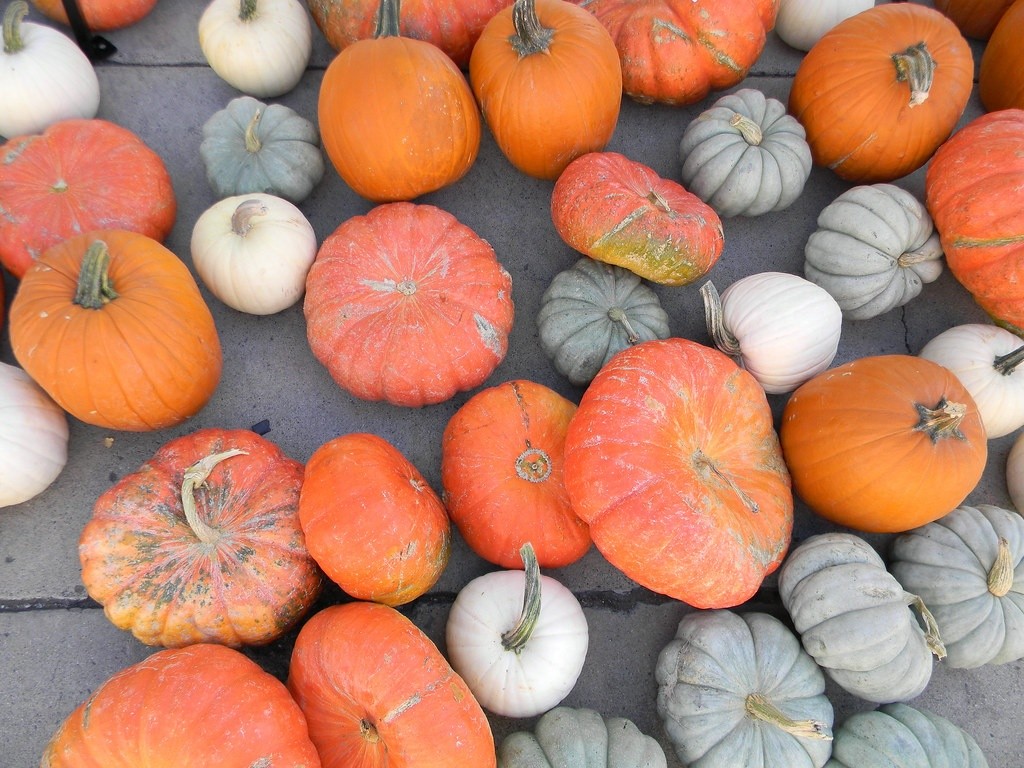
[0,0,1021,767]
